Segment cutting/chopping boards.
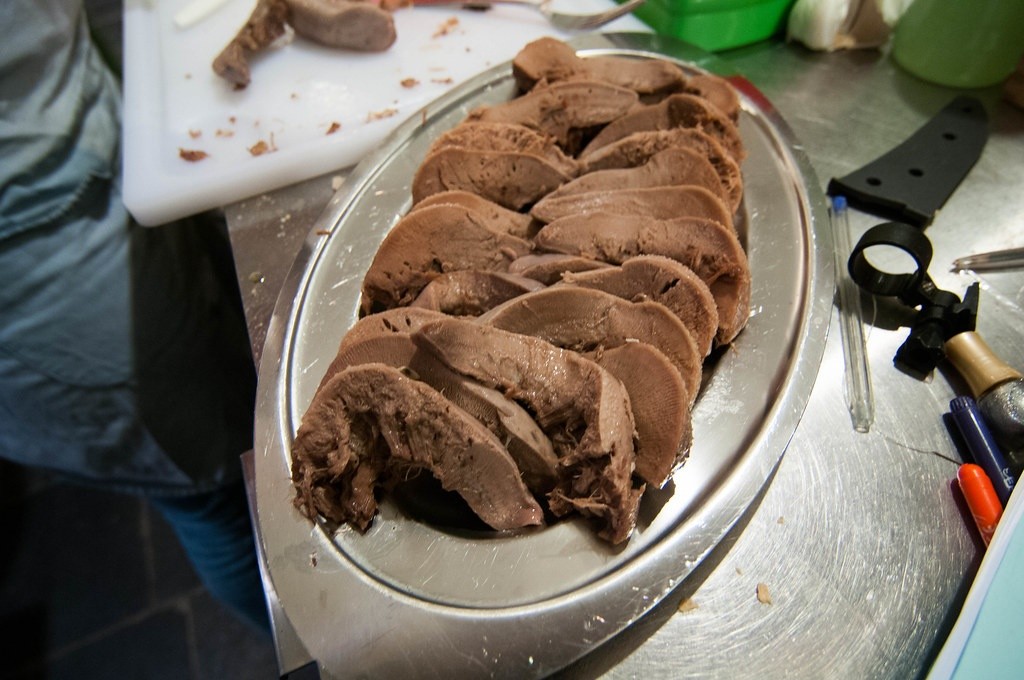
[123,0,657,227]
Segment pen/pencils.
[948,394,1018,549]
[953,246,1024,271]
[831,194,874,430]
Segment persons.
[0,0,274,641]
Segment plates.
[253,31,836,680]
